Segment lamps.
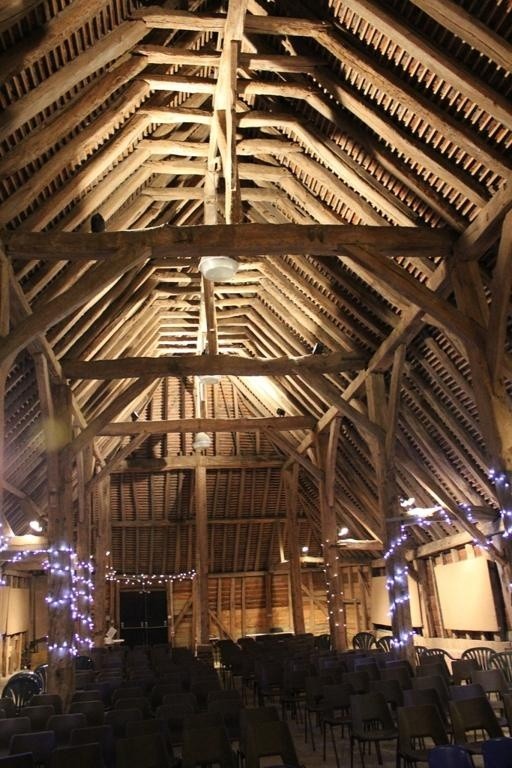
[196,249,238,285]
[192,428,213,450]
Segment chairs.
[0,620,511,767]
